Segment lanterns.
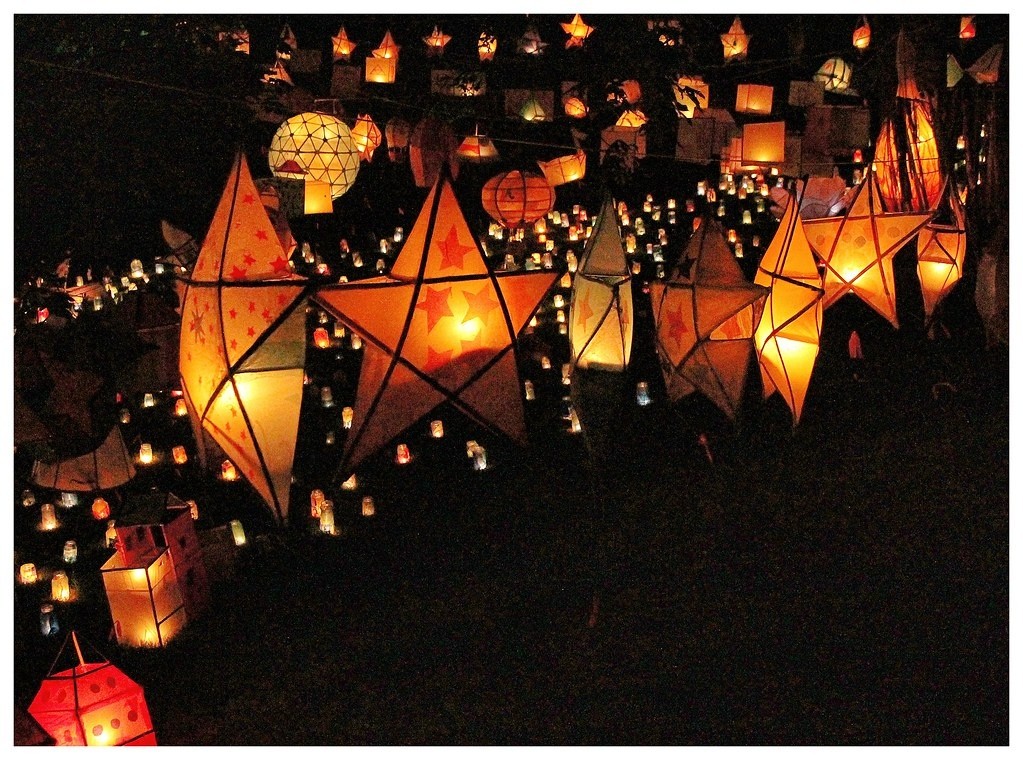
[13,12,1008,747]
